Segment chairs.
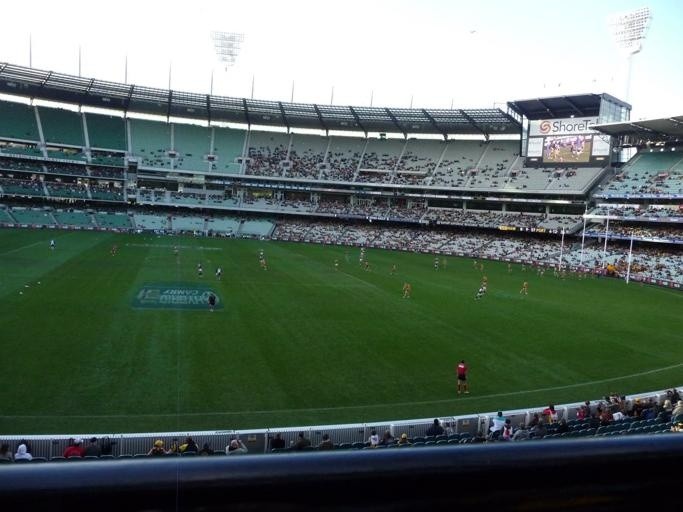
[487,411,674,453]
[332,432,487,452]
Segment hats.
[74,439,83,444]
[154,440,163,445]
[675,400,683,407]
[635,397,641,402]
[231,440,239,448]
[401,433,408,439]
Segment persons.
[518,280,529,295]
[401,279,411,299]
[206,292,216,313]
[473,284,486,302]
[456,359,470,394]
